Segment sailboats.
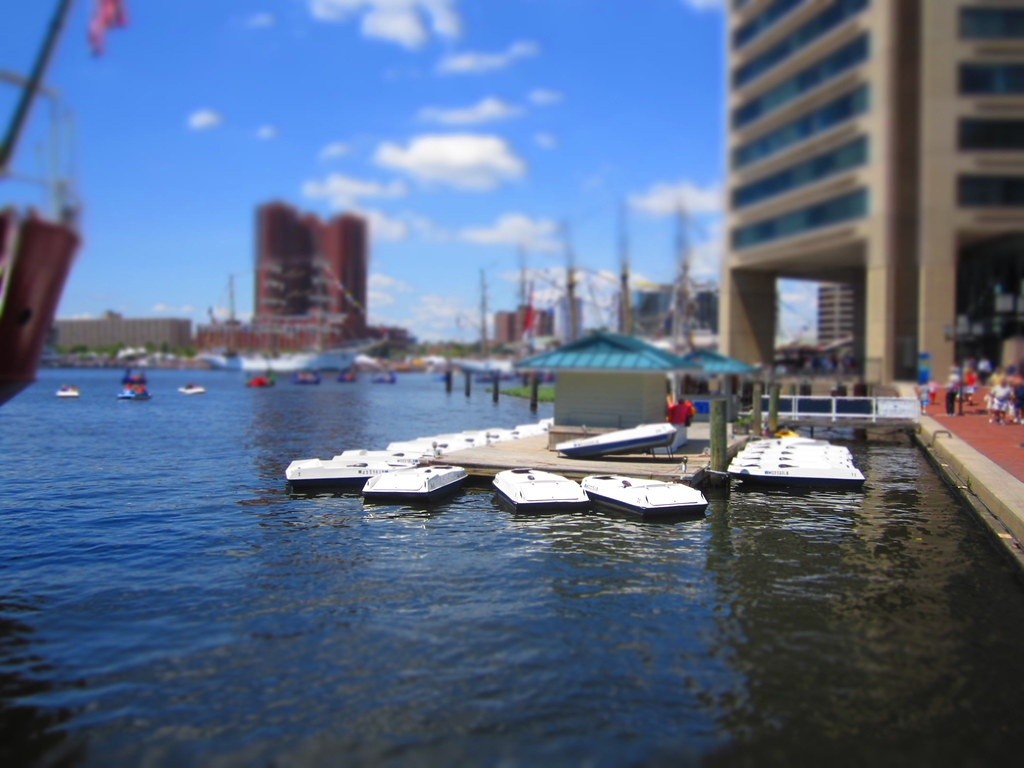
[2,0,131,408]
[195,256,389,372]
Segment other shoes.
[922,410,926,414]
[1013,418,1018,423]
[1020,419,1024,424]
[989,418,992,423]
[1000,419,1004,424]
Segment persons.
[942,365,961,417]
[918,382,930,414]
[122,367,134,385]
[668,395,692,424]
[964,367,977,406]
[929,379,938,404]
[62,383,77,392]
[977,355,992,385]
[985,366,1024,426]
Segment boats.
[579,474,709,520]
[362,464,468,506]
[285,418,554,487]
[56,388,79,397]
[117,390,153,400]
[727,436,867,489]
[493,468,593,512]
[556,422,677,460]
